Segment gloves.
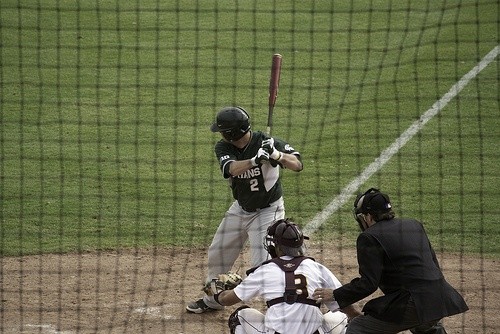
[251,148,270,166]
[262,137,283,162]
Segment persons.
[187,106,303,314]
[203,218,364,334]
[312,188,469,334]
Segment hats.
[364,192,391,212]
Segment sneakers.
[186,298,215,313]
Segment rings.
[318,295,321,298]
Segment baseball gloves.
[202,271,243,296]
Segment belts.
[240,205,270,213]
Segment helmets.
[211,107,250,142]
[274,222,303,247]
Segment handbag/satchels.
[361,296,399,319]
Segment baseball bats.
[259,53,282,164]
